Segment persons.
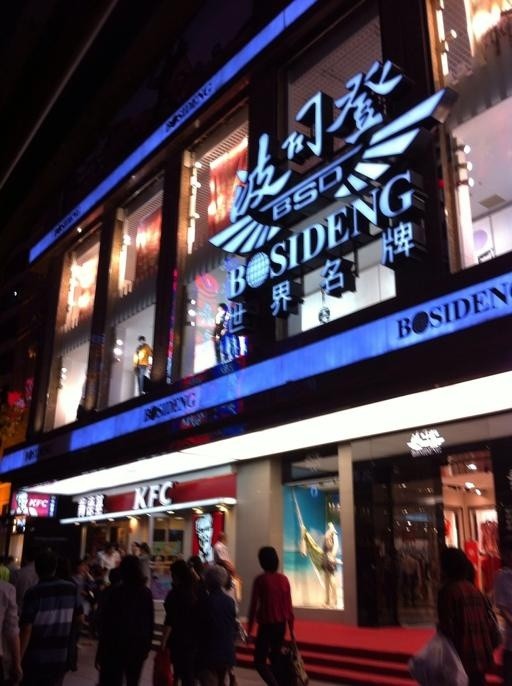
[433,545,503,686]
[321,520,340,607]
[246,545,298,685]
[227,334,241,360]
[132,333,155,395]
[9,490,39,517]
[19,552,84,686]
[193,513,217,568]
[0,538,154,650]
[494,564,512,686]
[91,552,156,686]
[0,576,24,685]
[210,302,230,366]
[160,554,249,685]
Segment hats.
[208,564,228,589]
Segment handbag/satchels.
[483,596,504,651]
[151,647,174,686]
[283,643,309,686]
